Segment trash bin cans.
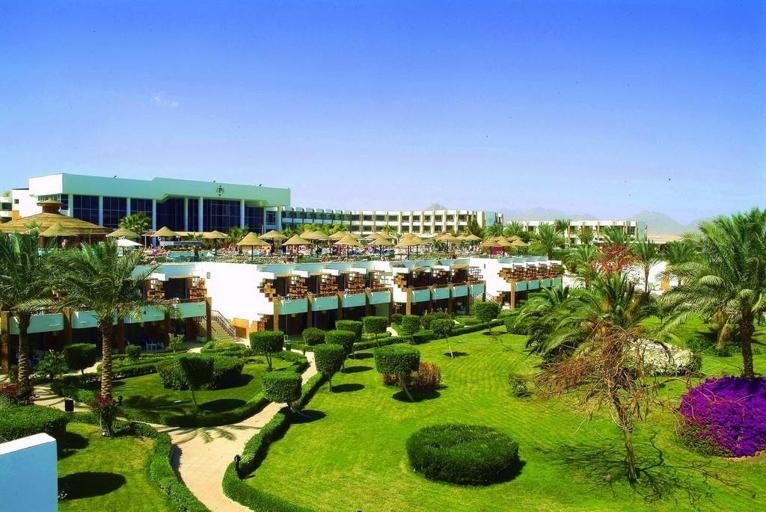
[65,400,74,412]
